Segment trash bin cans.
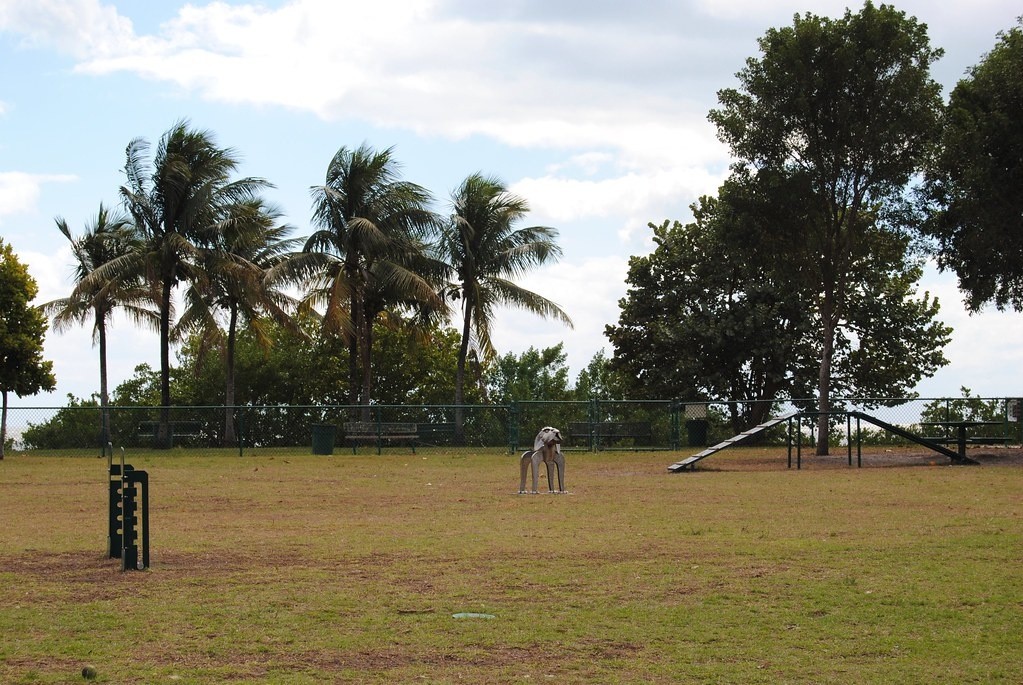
[310,423,338,456]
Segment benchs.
[568,420,654,445]
[137,420,202,447]
[342,422,419,455]
[414,421,455,445]
